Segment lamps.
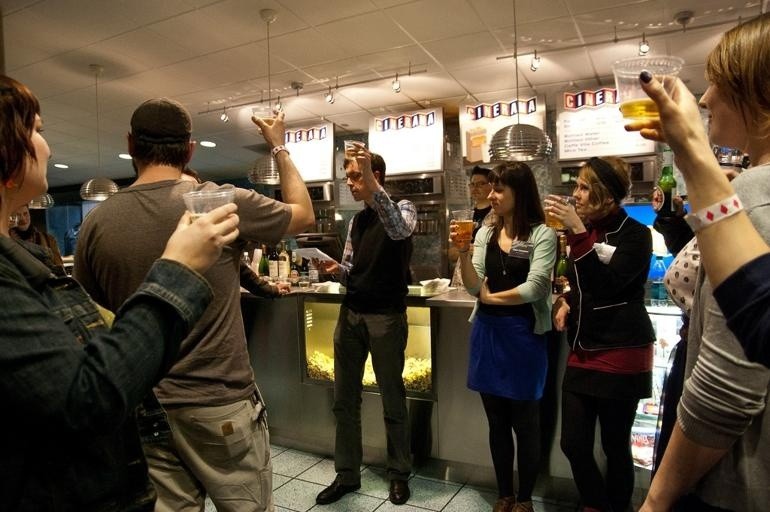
[246,9,284,186]
[79,66,121,202]
[488,1,553,165]
[27,193,54,210]
[218,71,405,125]
[529,33,654,72]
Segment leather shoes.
[390,480,410,505]
[493,496,516,512]
[316,474,361,504]
[512,498,535,512]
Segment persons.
[238,242,333,300]
[11,204,64,273]
[74,96,321,512]
[622,10,769,512]
[447,160,557,512]
[1,76,244,512]
[453,165,497,244]
[311,140,419,505]
[543,155,656,511]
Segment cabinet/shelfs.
[630,308,684,471]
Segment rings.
[561,199,568,206]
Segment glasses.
[467,181,487,187]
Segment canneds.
[414,219,438,234]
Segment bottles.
[269,239,279,277]
[713,143,750,169]
[308,258,319,283]
[657,146,677,218]
[258,244,269,276]
[278,240,291,277]
[556,234,570,293]
[300,256,309,276]
[243,252,251,263]
[650,255,668,306]
[304,209,332,233]
[290,248,299,286]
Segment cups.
[8,212,18,228]
[279,277,291,292]
[480,143,491,164]
[298,276,310,289]
[253,106,274,137]
[545,194,576,230]
[452,208,474,243]
[344,139,365,162]
[613,55,685,121]
[182,190,236,223]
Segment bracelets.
[456,246,473,254]
[265,142,292,161]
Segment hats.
[131,96,191,143]
